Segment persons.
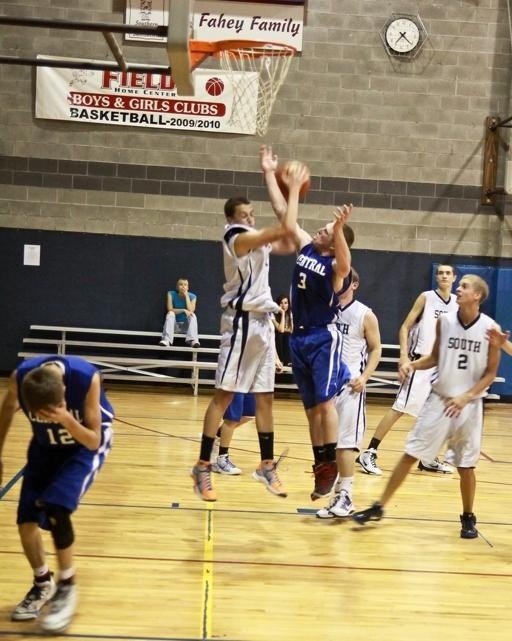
[485,328,512,359]
[2,354,114,629]
[260,144,355,500]
[269,294,294,366]
[316,266,382,518]
[351,271,501,539]
[159,277,202,348]
[211,352,283,474]
[355,263,456,473]
[191,166,308,502]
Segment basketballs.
[275,161,311,200]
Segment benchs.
[18,323,506,404]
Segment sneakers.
[311,461,339,500]
[316,489,353,518]
[354,500,383,522]
[192,459,216,501]
[252,460,287,498]
[213,453,241,475]
[355,448,382,475]
[12,576,58,621]
[460,513,478,538]
[418,457,452,474]
[40,575,77,632]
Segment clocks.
[378,11,428,59]
